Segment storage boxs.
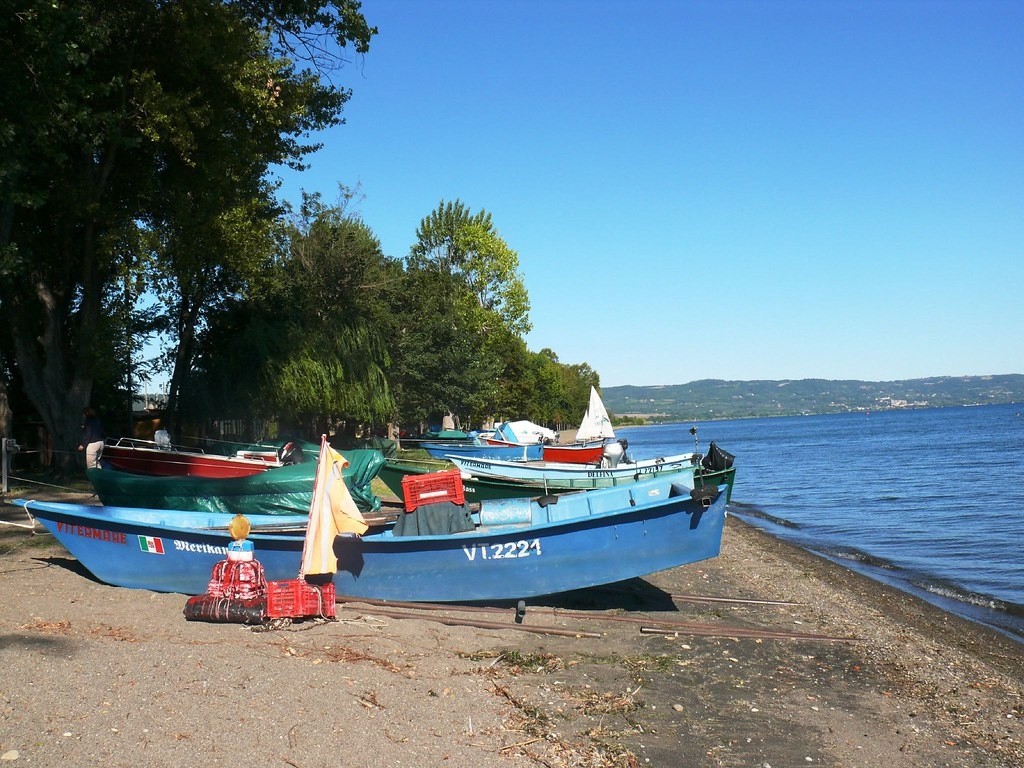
[229,541,255,562]
[401,468,464,512]
[267,581,335,618]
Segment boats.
[4,443,728,607]
[84,423,711,515]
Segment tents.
[492,420,555,443]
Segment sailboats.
[575,386,617,447]
